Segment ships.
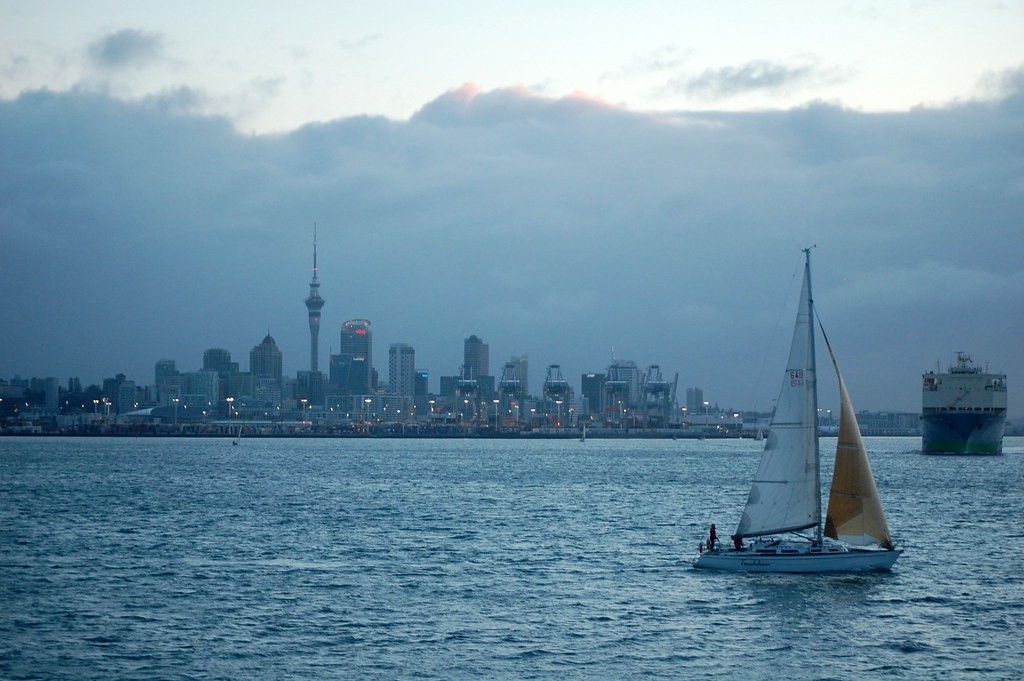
[920,351,1009,456]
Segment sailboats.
[693,244,908,576]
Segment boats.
[3,393,775,443]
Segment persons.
[734,538,744,551]
[710,523,718,551]
